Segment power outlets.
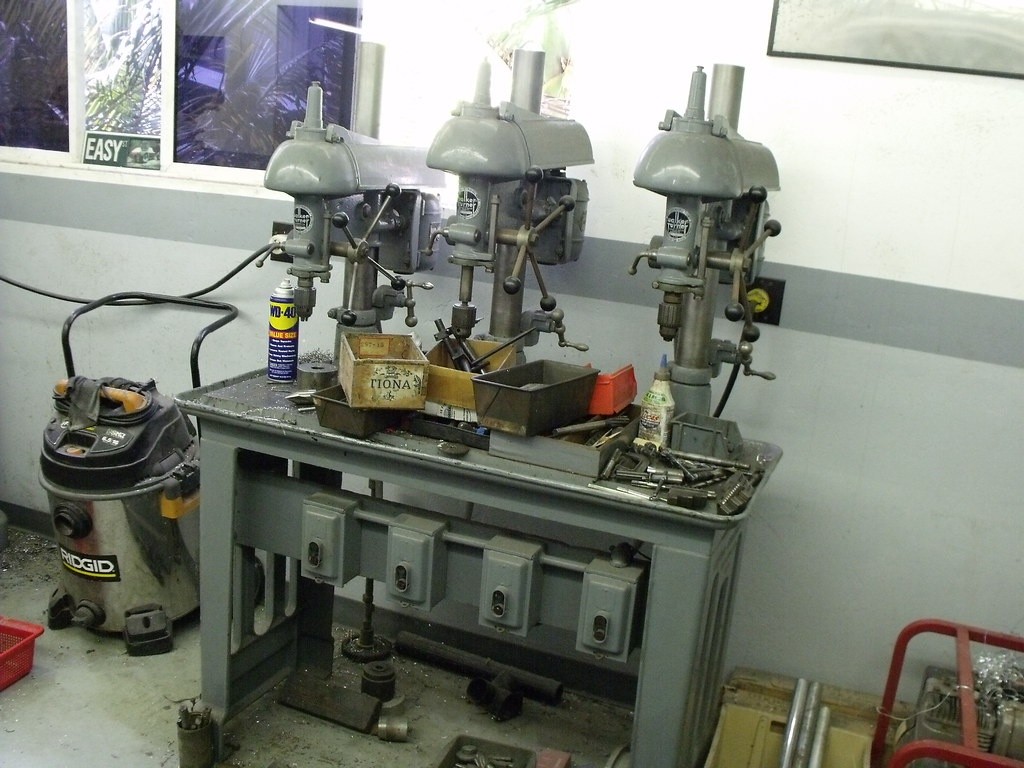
[738,273,787,325]
[269,223,291,263]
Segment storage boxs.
[339,331,426,412]
[424,339,516,413]
[0,617,44,689]
[589,364,637,415]
[705,703,874,768]
[311,384,396,438]
[470,359,601,436]
[487,403,642,478]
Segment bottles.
[638,354,674,455]
[268,278,298,384]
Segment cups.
[177,711,213,768]
